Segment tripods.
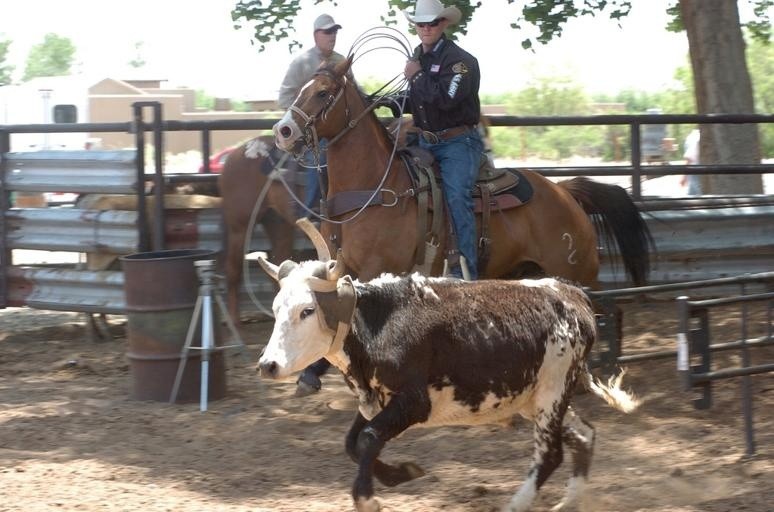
[166,257,256,414]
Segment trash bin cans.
[120,248,225,400]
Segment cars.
[197,147,238,176]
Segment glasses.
[322,28,338,34]
[415,20,441,27]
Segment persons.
[377,0,482,280]
[277,14,348,224]
[681,127,702,197]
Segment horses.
[214,105,495,327]
[271,53,658,291]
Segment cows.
[259,248,642,512]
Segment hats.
[312,14,342,32]
[403,0,463,24]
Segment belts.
[415,125,469,145]
